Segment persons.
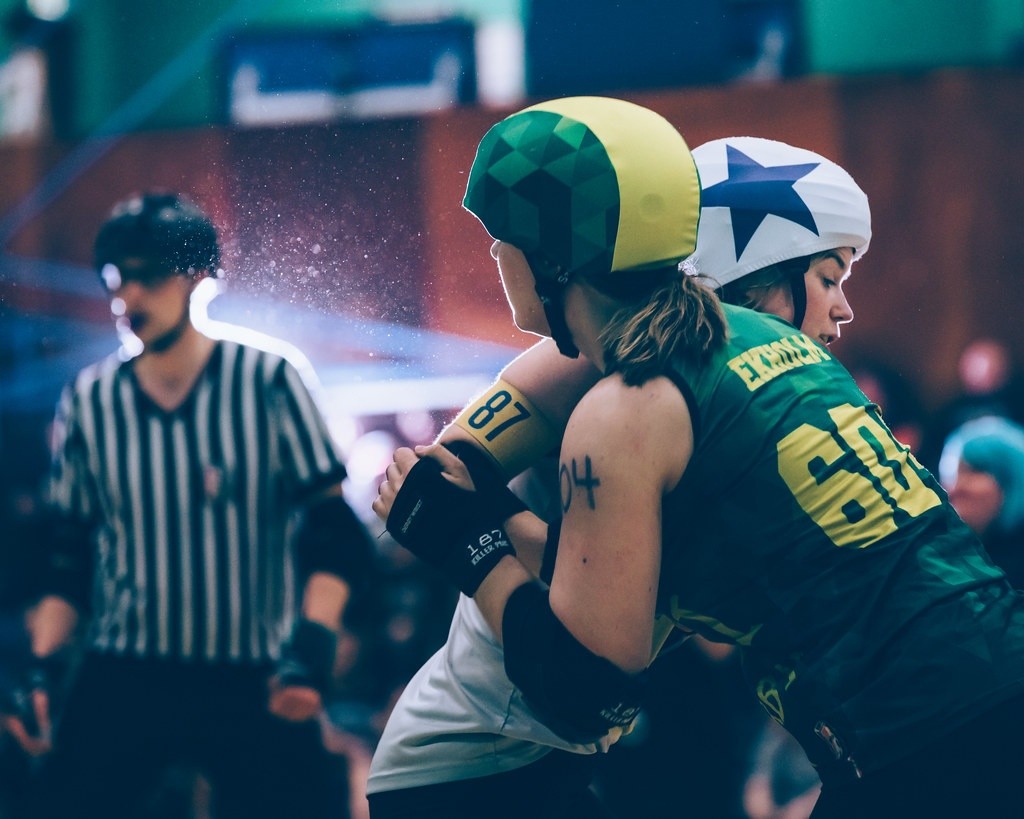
[937,416,1024,547]
[372,96,1024,819]
[2,187,372,819]
[359,137,871,819]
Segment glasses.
[104,263,179,291]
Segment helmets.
[464,95,700,271]
[686,137,873,287]
[89,195,219,272]
